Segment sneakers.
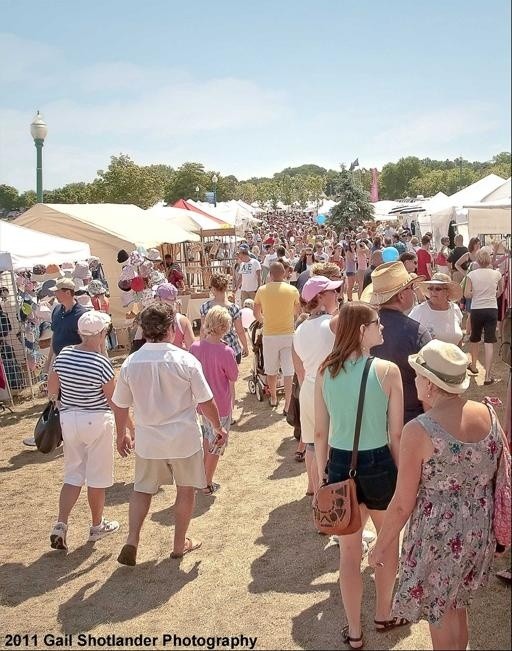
[88,518,121,543]
[294,443,308,464]
[48,522,71,553]
[23,436,37,447]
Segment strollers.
[247,319,298,402]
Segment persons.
[23,212,512,651]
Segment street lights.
[29,109,50,205]
[211,174,219,208]
[195,185,200,202]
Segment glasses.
[364,317,381,327]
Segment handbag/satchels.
[481,393,512,550]
[433,246,452,267]
[312,478,364,540]
[34,394,64,456]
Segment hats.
[405,338,473,397]
[12,244,185,373]
[299,275,345,305]
[411,272,465,305]
[357,259,421,307]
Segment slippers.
[116,544,138,568]
[169,536,203,560]
[466,362,479,375]
[283,407,289,417]
[268,396,278,408]
[483,378,495,386]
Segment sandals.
[494,567,512,586]
[202,482,222,497]
[373,615,410,634]
[340,623,364,651]
